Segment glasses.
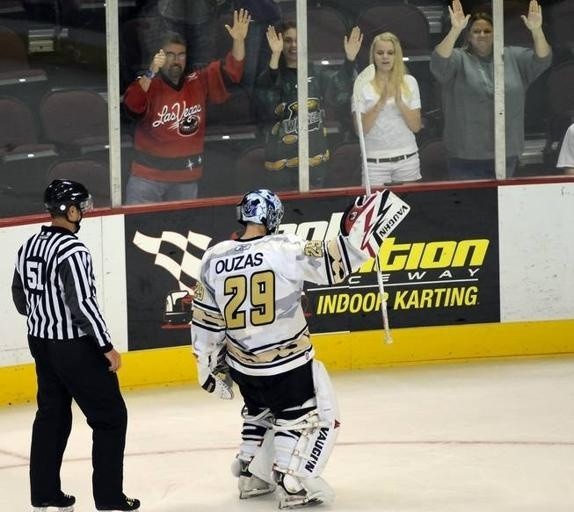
[164,53,185,61]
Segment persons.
[349,32,423,187]
[250,18,363,192]
[11,176,140,511]
[190,188,413,510]
[555,122,573,175]
[123,6,253,203]
[428,0,555,180]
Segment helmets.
[43,177,89,216]
[236,187,283,225]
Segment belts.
[366,150,418,163]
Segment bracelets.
[146,69,156,80]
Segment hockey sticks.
[352,64,393,343]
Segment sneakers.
[94,494,140,511]
[30,491,76,507]
[235,468,271,491]
[276,473,311,499]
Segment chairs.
[3,2,574,206]
